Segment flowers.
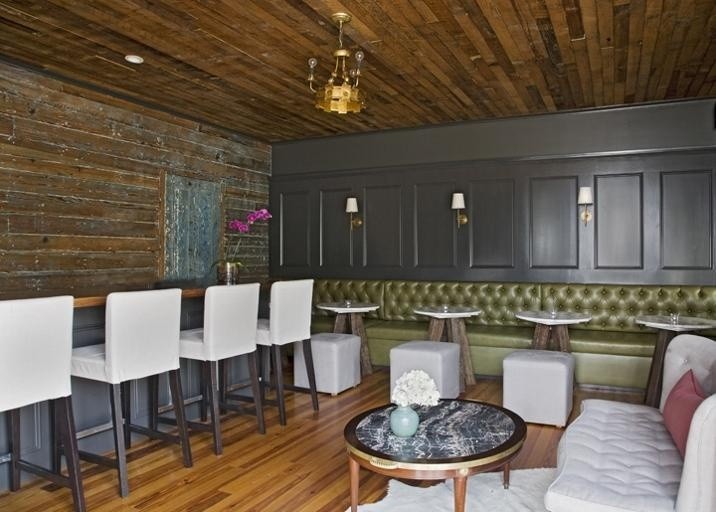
[390,369,440,407]
[210,209,272,273]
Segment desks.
[413,306,483,385]
[316,303,382,373]
[73,280,207,309]
[634,313,716,406]
[515,311,594,396]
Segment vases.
[390,407,419,438]
[217,262,239,281]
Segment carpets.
[346,467,556,512]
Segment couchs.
[543,334,716,512]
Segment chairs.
[150,280,267,455]
[222,280,319,425]
[51,287,195,498]
[2,293,86,511]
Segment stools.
[502,350,574,428]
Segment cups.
[669,312,680,323]
[343,299,353,307]
[549,306,558,316]
[441,303,450,313]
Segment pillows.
[664,368,708,460]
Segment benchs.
[541,280,716,396]
[300,279,386,366]
[366,283,540,378]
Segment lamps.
[578,187,593,226]
[346,198,363,230]
[451,193,468,229]
[307,13,368,114]
